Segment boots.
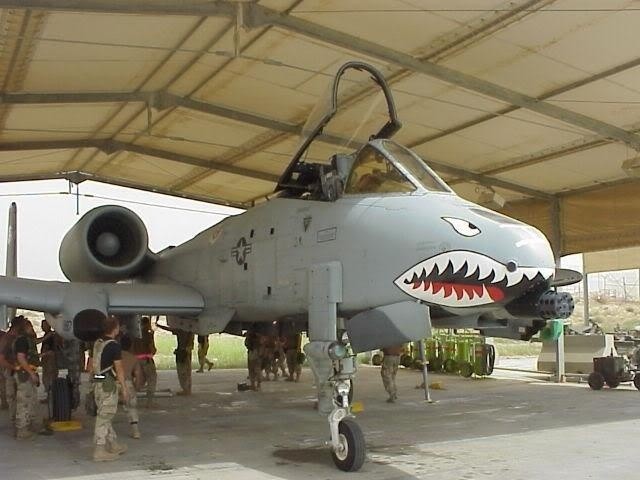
[129,423,141,439]
[386,395,396,402]
[208,363,213,371]
[92,444,119,462]
[109,440,128,455]
[38,428,53,435]
[176,387,190,396]
[196,365,203,372]
[16,423,38,441]
[247,368,301,391]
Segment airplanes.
[0,60,582,473]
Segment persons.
[156,324,194,395]
[382,344,401,402]
[197,333,215,373]
[115,338,143,439]
[87,316,127,462]
[0,316,56,441]
[243,328,305,392]
[134,317,159,400]
